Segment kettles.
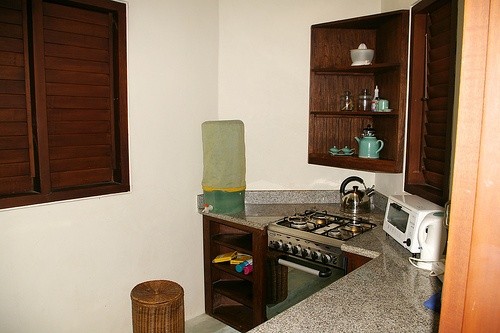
[416,211,448,263]
[339,176,375,215]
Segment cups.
[341,146,350,153]
[378,99,389,112]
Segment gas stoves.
[266,208,378,267]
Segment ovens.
[264,251,346,316]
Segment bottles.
[357,89,373,111]
[361,124,376,137]
[340,90,354,111]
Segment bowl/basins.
[329,145,339,153]
[350,49,374,63]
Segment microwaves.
[382,193,446,256]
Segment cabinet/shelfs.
[203,215,273,333]
[308,8,410,173]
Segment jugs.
[354,135,384,159]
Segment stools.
[130,280,185,333]
